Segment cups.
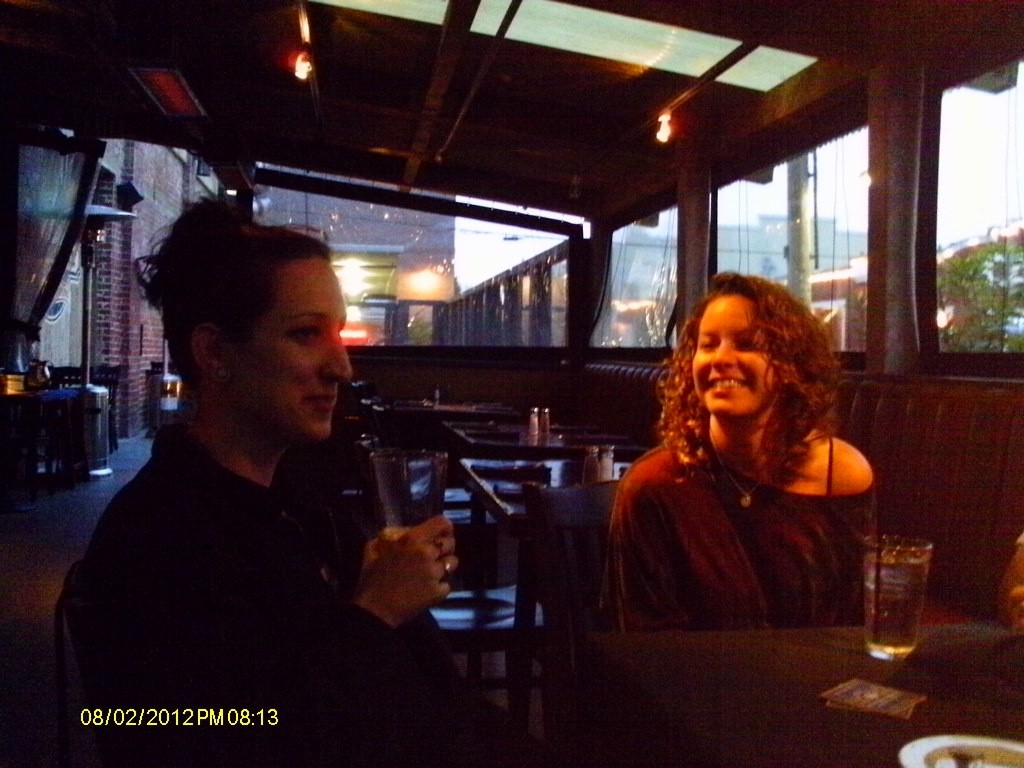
[369,449,449,533]
[862,533,932,660]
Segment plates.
[898,733,1024,768]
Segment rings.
[445,562,451,573]
[433,539,443,553]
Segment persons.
[49,195,462,767]
[998,531,1024,630]
[601,272,878,634]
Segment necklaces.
[714,452,764,507]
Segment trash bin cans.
[66,385,108,470]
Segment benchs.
[581,362,1024,628]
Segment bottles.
[528,407,539,436]
[598,444,616,483]
[23,359,52,390]
[582,446,601,485]
[539,408,550,435]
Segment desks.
[459,456,633,731]
[0,390,54,478]
[589,631,1024,768]
[441,419,647,452]
[397,397,521,418]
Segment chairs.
[146,362,163,428]
[348,380,545,732]
[53,559,104,768]
[48,363,122,454]
